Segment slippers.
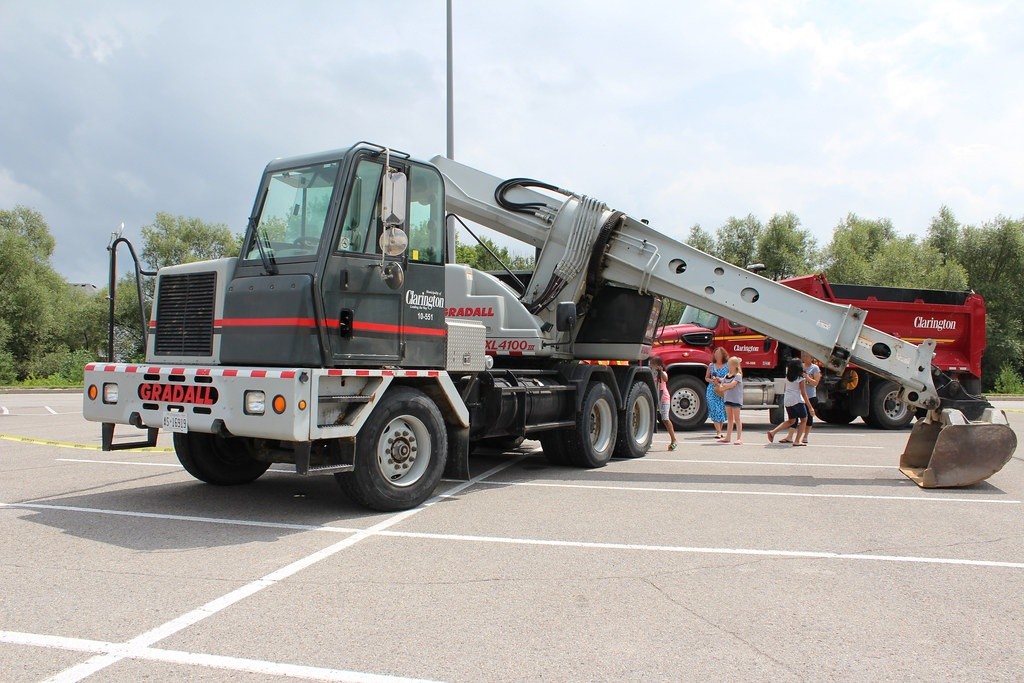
[802,438,808,443]
[779,438,793,443]
[768,431,774,442]
[793,441,806,446]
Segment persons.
[767,357,815,446]
[648,356,677,451]
[779,350,821,443]
[705,347,729,438]
[717,356,743,444]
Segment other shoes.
[668,442,678,451]
[721,435,725,438]
[734,439,742,445]
[715,435,720,438]
[718,438,730,442]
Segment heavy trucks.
[83,140,1020,513]
[641,270,989,433]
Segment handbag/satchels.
[715,383,725,398]
[806,364,821,387]
